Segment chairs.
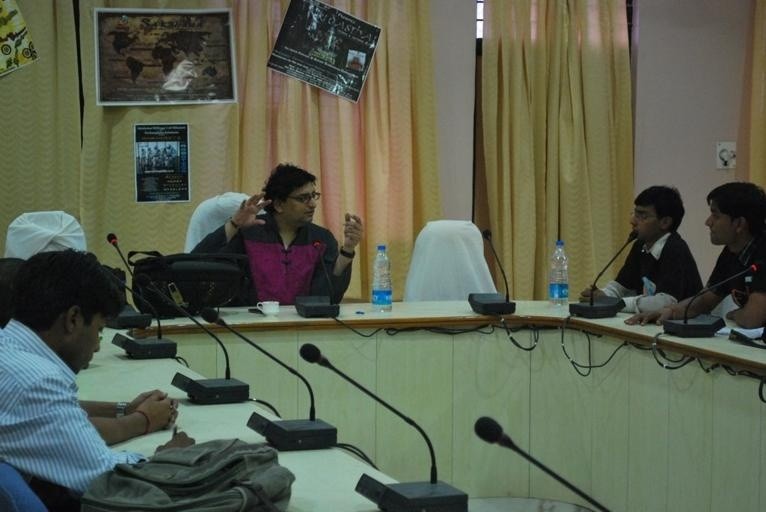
[401,220,500,306]
[4,210,88,263]
[181,192,266,255]
[3,464,58,512]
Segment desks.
[70,279,766,511]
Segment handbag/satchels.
[80,439,295,512]
[132,252,243,316]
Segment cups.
[256,300,280,317]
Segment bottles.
[547,242,570,318]
[371,245,393,313]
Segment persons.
[577,182,705,315]
[189,162,364,305]
[624,180,766,332]
[0,248,196,512]
[76,387,179,447]
[136,142,177,172]
[0,257,25,333]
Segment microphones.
[201,308,336,452]
[85,251,177,360]
[474,416,610,512]
[137,273,249,405]
[569,230,638,319]
[468,229,515,315]
[104,232,152,329]
[299,343,468,512]
[663,258,764,338]
[295,231,340,318]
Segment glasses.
[287,192,320,203]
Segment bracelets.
[114,401,127,422]
[339,245,355,259]
[133,408,149,435]
[228,215,240,230]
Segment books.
[717,327,764,339]
